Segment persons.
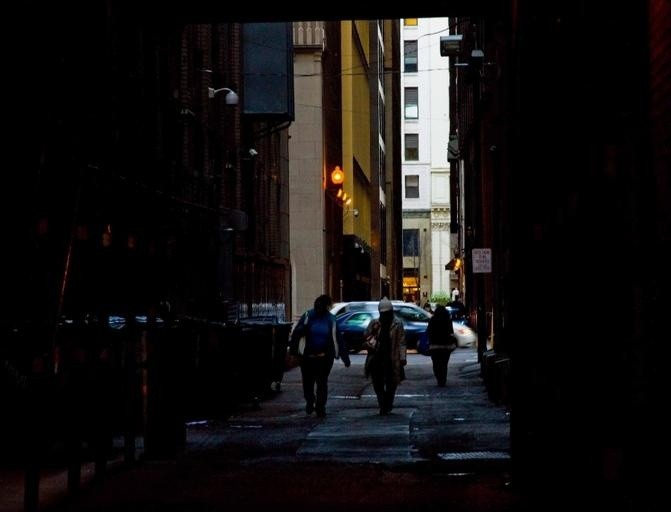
[361,304,407,414]
[426,306,452,387]
[287,295,350,416]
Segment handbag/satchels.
[361,335,378,349]
[419,333,430,356]
[291,321,306,355]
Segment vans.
[327,298,476,351]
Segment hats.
[379,297,393,312]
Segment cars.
[333,310,433,356]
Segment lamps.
[208,86,241,106]
